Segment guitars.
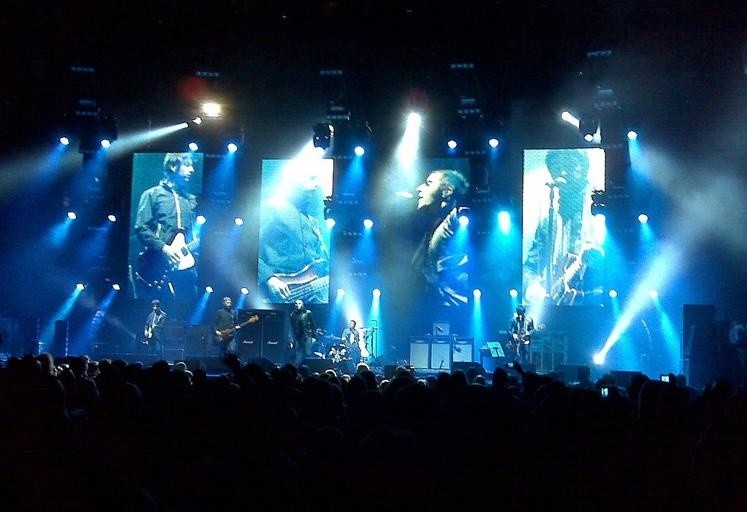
[507,323,545,350]
[145,314,162,338]
[138,228,200,297]
[214,315,259,346]
[541,247,597,306]
[259,259,329,304]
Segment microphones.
[519,315,523,322]
[551,177,567,190]
[359,326,366,330]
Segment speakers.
[300,357,334,374]
[683,303,747,393]
[408,320,475,369]
[492,363,536,387]
[555,364,590,381]
[609,370,650,389]
[452,362,487,382]
[383,364,400,378]
[236,309,296,366]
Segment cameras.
[660,375,670,383]
[600,386,609,398]
[507,362,515,368]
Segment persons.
[141,299,167,364]
[286,300,318,369]
[505,305,534,366]
[257,162,329,305]
[328,346,340,364]
[408,167,471,305]
[1,349,747,512]
[211,296,241,357]
[132,153,199,302]
[522,147,605,307]
[340,319,361,366]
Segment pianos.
[323,336,343,345]
